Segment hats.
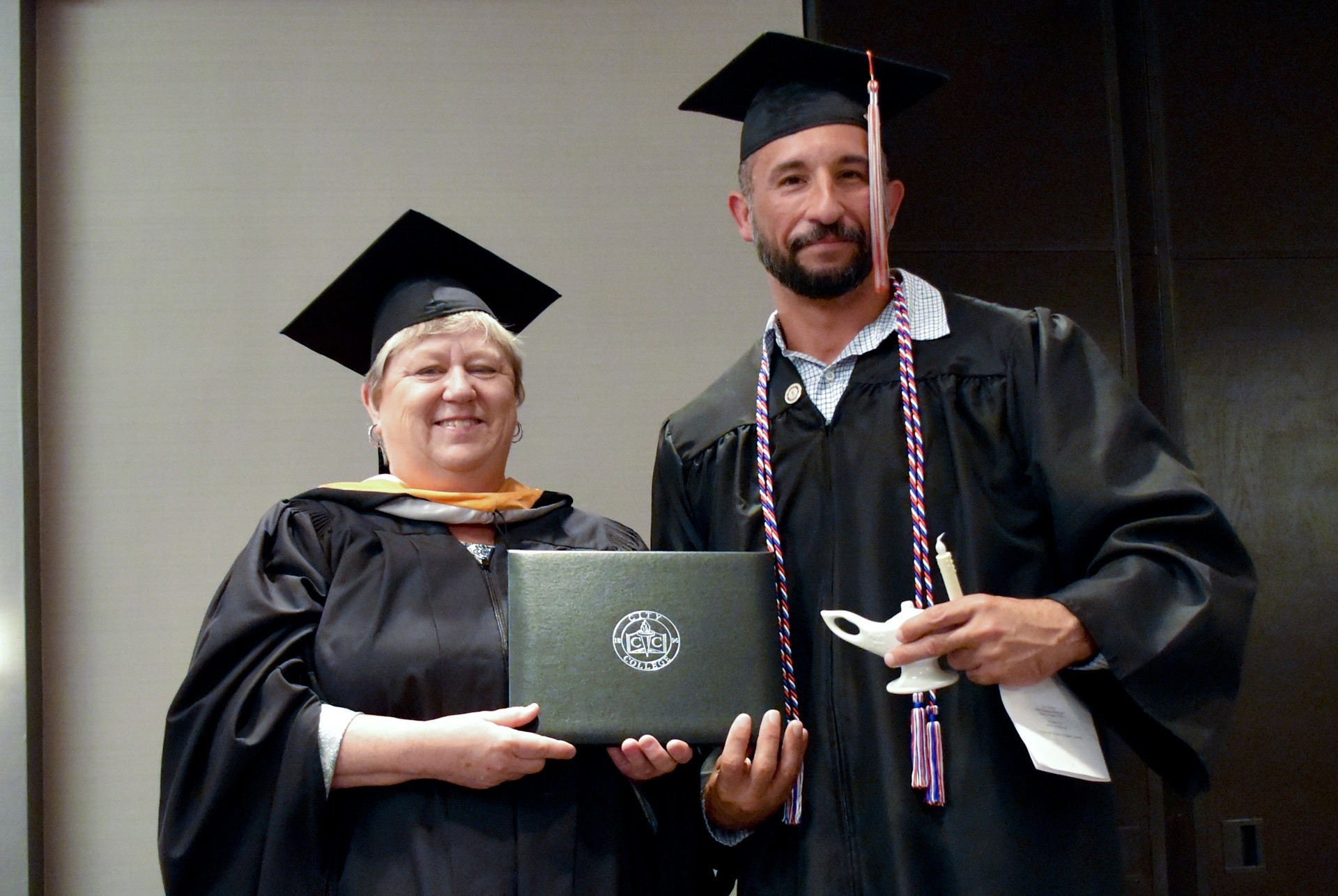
[281,207,562,375]
[675,29,950,295]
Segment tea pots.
[821,601,959,694]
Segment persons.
[156,203,691,896]
[645,31,1255,896]
